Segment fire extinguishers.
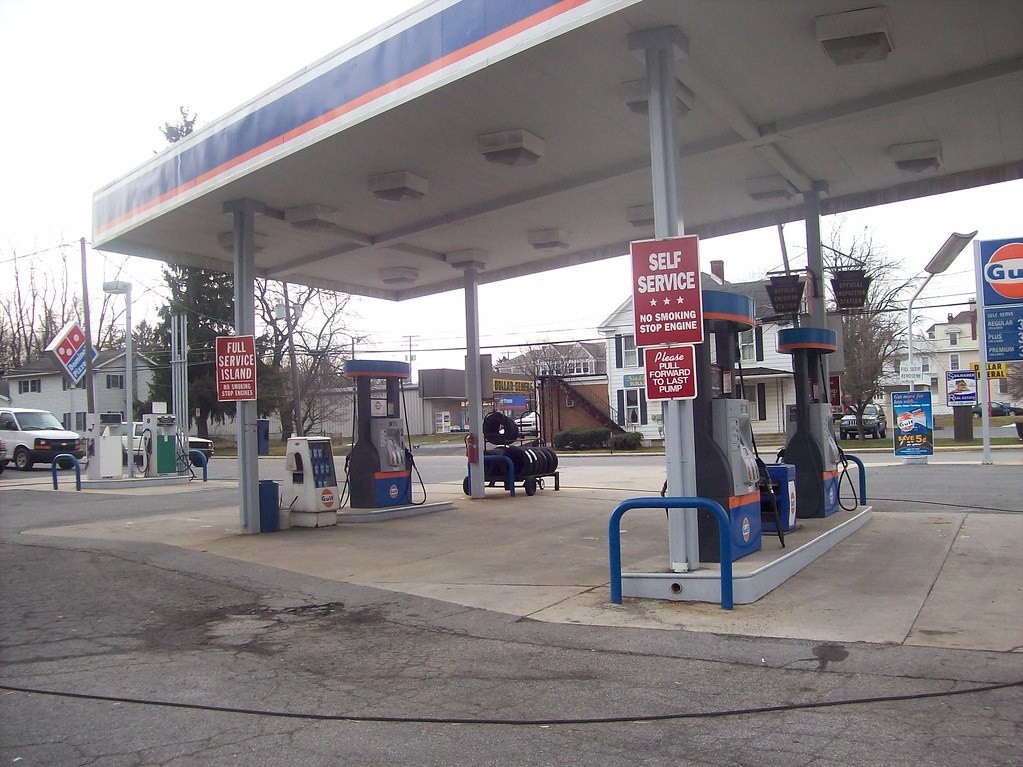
[464,432,478,463]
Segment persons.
[969,381,975,387]
[951,380,970,393]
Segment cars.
[98,421,215,467]
[972,401,1023,416]
[513,410,538,436]
[0,438,9,474]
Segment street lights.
[907,231,979,391]
[275,302,304,437]
[103,280,136,479]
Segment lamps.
[285,203,342,233]
[446,250,489,271]
[890,140,944,175]
[369,172,429,204]
[625,77,694,120]
[742,175,797,209]
[625,204,654,227]
[480,128,545,167]
[379,267,421,289]
[814,7,897,69]
[528,229,572,254]
[217,232,269,257]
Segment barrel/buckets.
[280,506,290,530]
[258,479,281,532]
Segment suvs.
[0,407,84,472]
[838,403,888,439]
